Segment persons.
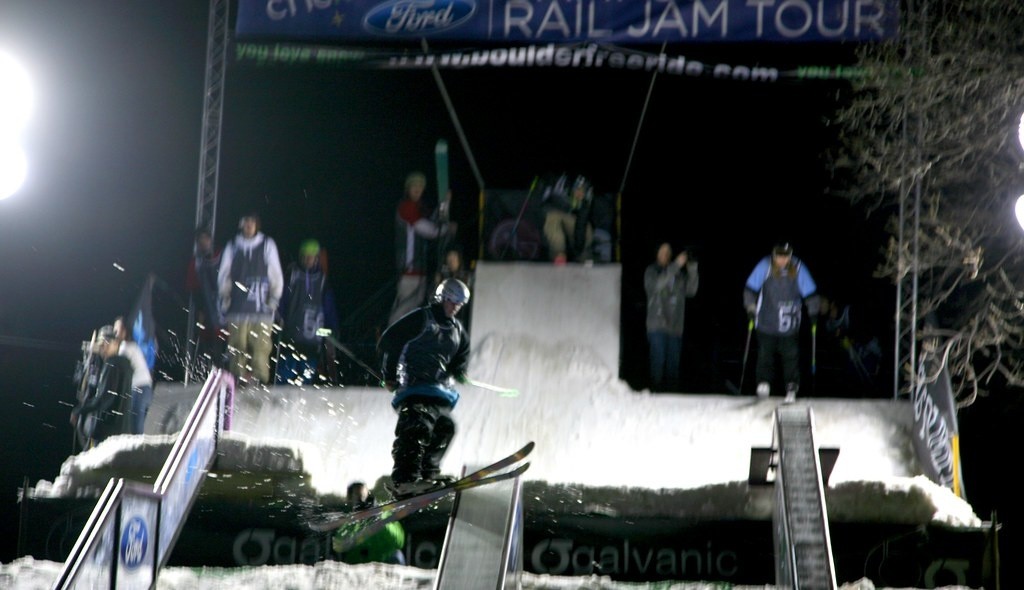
[393,159,598,372]
[71,314,150,452]
[744,242,820,395]
[813,293,887,397]
[193,217,335,387]
[331,483,406,569]
[378,278,472,498]
[644,245,700,392]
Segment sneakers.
[430,474,457,485]
[393,472,444,501]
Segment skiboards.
[308,439,535,552]
[757,388,798,404]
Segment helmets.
[404,171,426,190]
[772,238,793,258]
[434,277,470,304]
[237,208,261,228]
[298,238,320,257]
